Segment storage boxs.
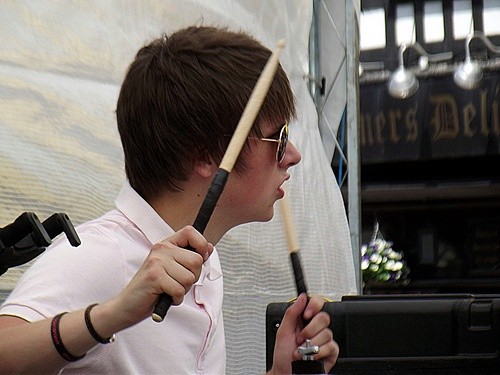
[266,292,500,375]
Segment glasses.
[221,123,289,164]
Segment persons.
[0,26,339,375]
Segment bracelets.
[85,302,115,344]
[51,311,86,361]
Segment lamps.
[387,42,452,99]
[453,31,500,90]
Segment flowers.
[359,240,408,282]
